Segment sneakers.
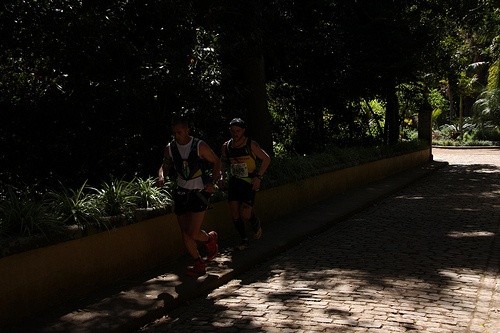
[185,258,206,278]
[249,214,262,239]
[203,229,219,260]
[236,236,251,251]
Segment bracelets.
[210,179,215,185]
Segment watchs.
[257,175,263,179]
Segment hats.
[228,117,246,129]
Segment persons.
[220,118,269,250]
[158,117,221,276]
